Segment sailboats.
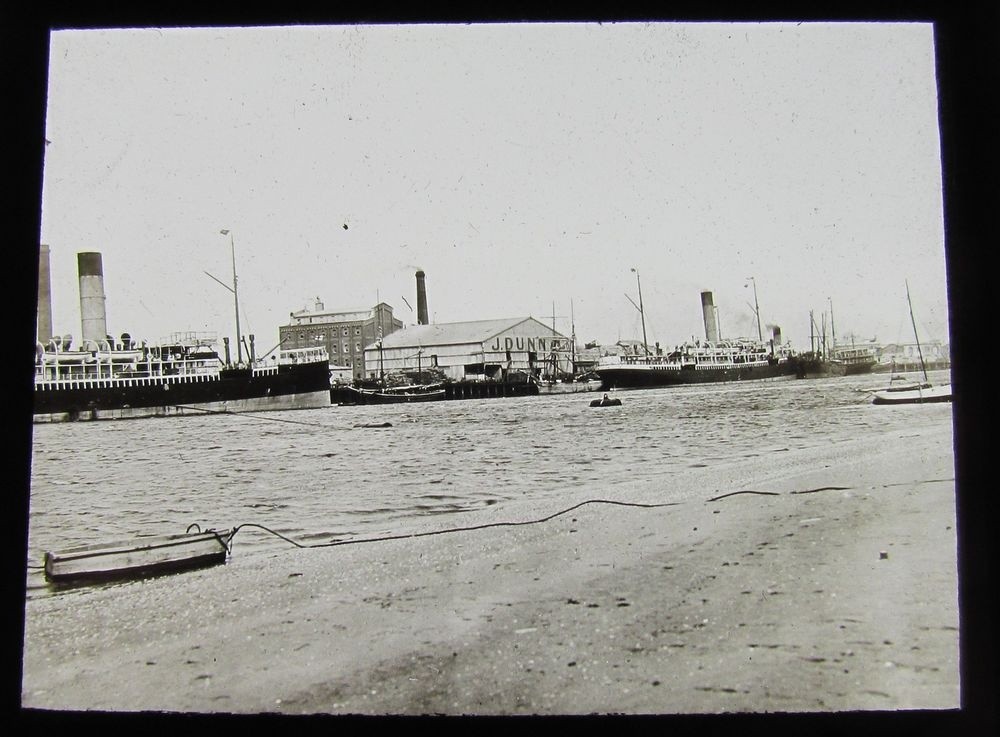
[33,230,338,422]
[595,268,799,389]
[332,298,602,404]
[803,298,877,380]
[871,277,952,405]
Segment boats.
[853,381,933,394]
[44,528,232,582]
